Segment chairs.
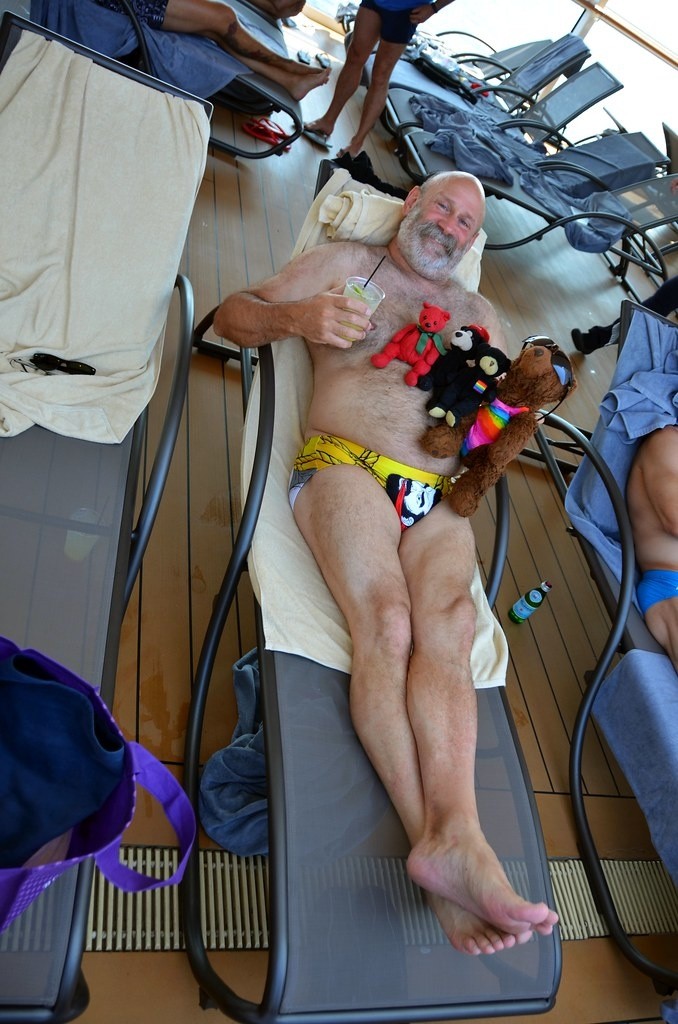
[0,0,678,1024]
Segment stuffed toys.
[370,301,577,519]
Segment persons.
[572,274,678,355]
[213,171,561,954]
[89,0,332,102]
[626,425,678,674]
[246,0,306,19]
[303,0,454,159]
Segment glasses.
[519,335,574,422]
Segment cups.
[335,276,385,342]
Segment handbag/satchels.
[0,634,197,928]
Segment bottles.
[508,580,552,624]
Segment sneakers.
[571,328,595,354]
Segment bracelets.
[431,3,439,13]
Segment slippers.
[242,122,291,150]
[292,121,333,149]
[250,118,290,139]
[316,52,331,68]
[297,50,311,65]
